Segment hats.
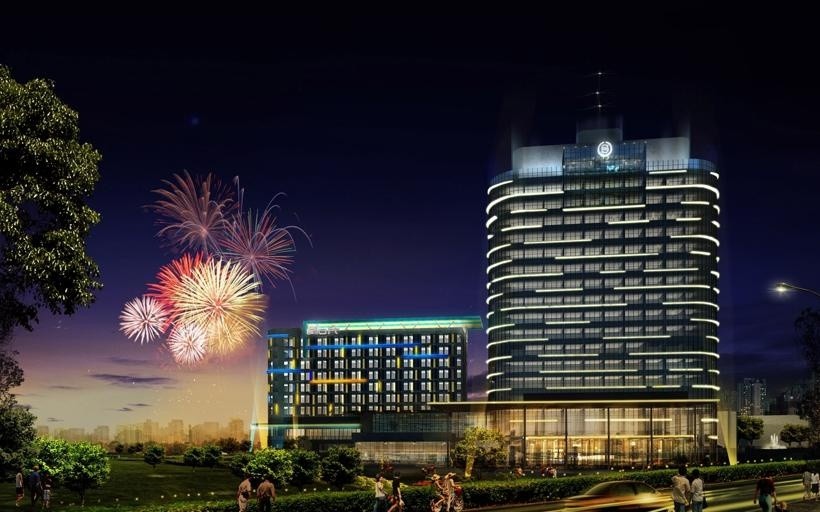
[444,472,456,479]
[430,473,440,481]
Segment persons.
[802,467,820,501]
[255,475,275,512]
[540,465,557,479]
[753,471,791,512]
[430,471,459,512]
[691,469,705,512]
[509,464,522,481]
[670,465,690,512]
[237,475,253,512]
[15,464,52,512]
[387,470,404,512]
[373,473,388,512]
